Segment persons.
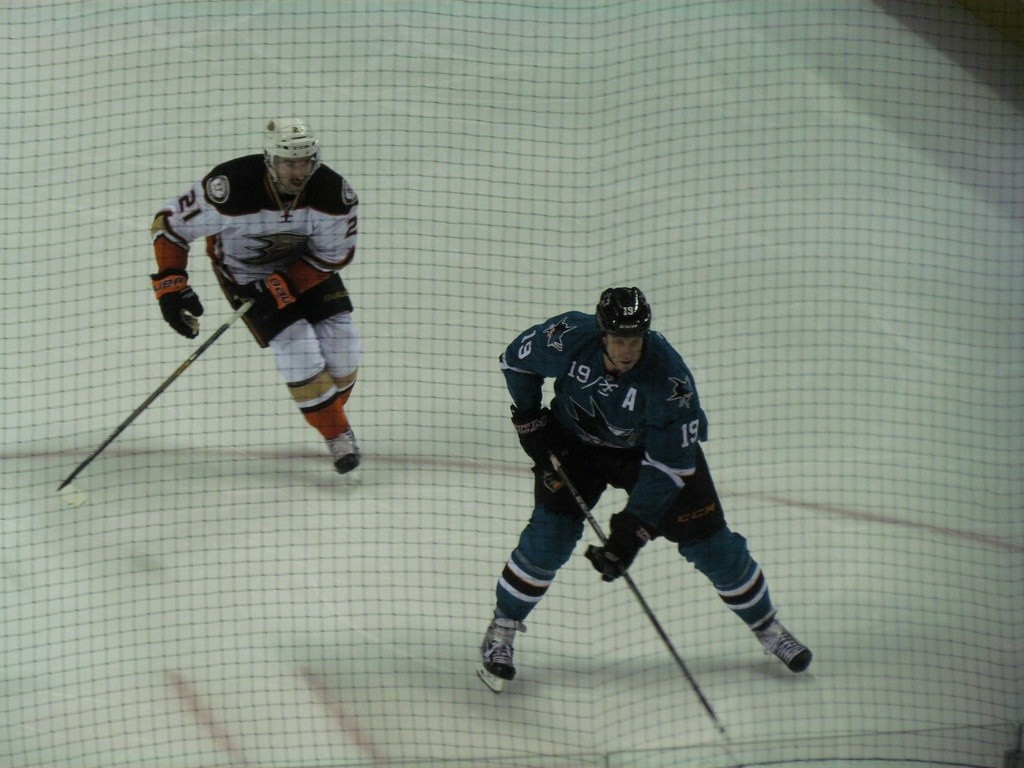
[146,118,371,480]
[474,288,814,693]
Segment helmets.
[263,117,320,169]
[595,287,654,337]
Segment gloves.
[584,512,651,583]
[509,404,558,463]
[234,270,298,329]
[150,268,204,340]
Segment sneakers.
[476,611,527,692]
[326,426,361,481]
[754,620,813,674]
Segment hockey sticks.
[551,450,736,748]
[53,298,252,494]
